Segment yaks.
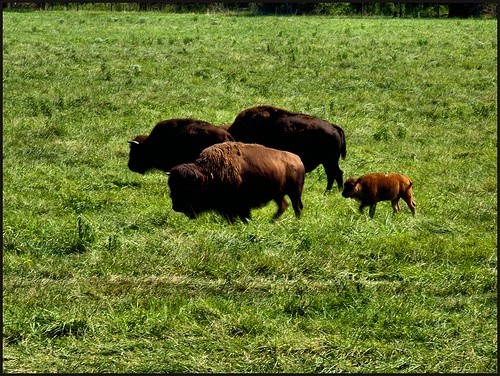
[342,174,416,216]
[127,117,235,175]
[168,141,305,226]
[227,106,347,192]
